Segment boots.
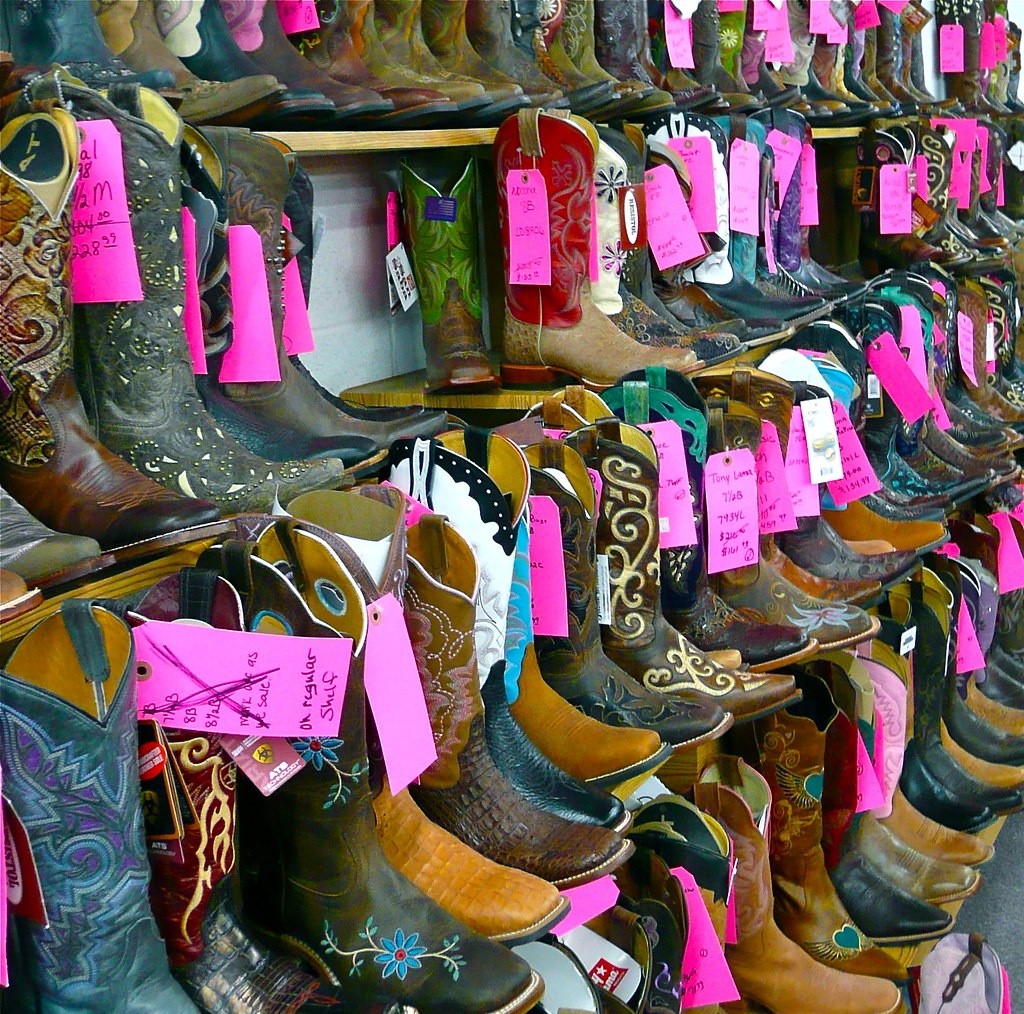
[2,1,1024,1014]
